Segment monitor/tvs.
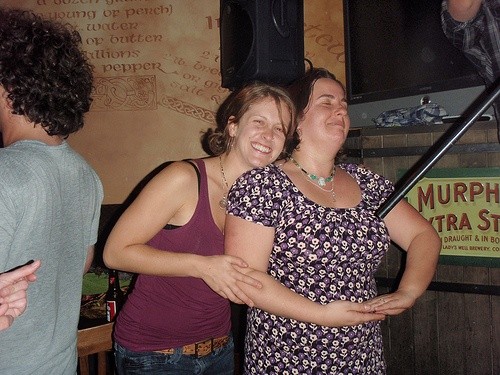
[342,0,487,104]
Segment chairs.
[78,320,116,375]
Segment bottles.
[105,269,125,323]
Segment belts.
[153,336,228,358]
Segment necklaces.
[219,154,230,207]
[288,154,337,202]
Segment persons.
[103,81,297,375]
[441,0,500,144]
[223,68,442,375]
[0,259,41,331]
[0,8,105,375]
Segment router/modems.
[441,114,496,123]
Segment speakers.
[220,0,304,92]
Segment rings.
[380,298,386,303]
